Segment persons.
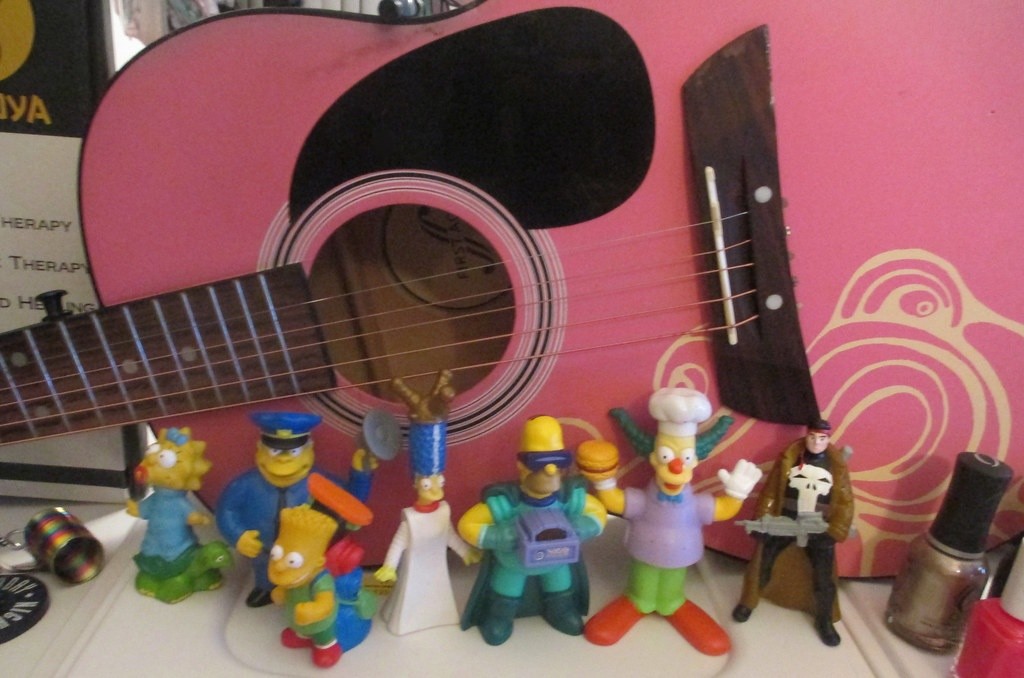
[731,418,856,648]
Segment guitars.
[0,0,1023,582]
